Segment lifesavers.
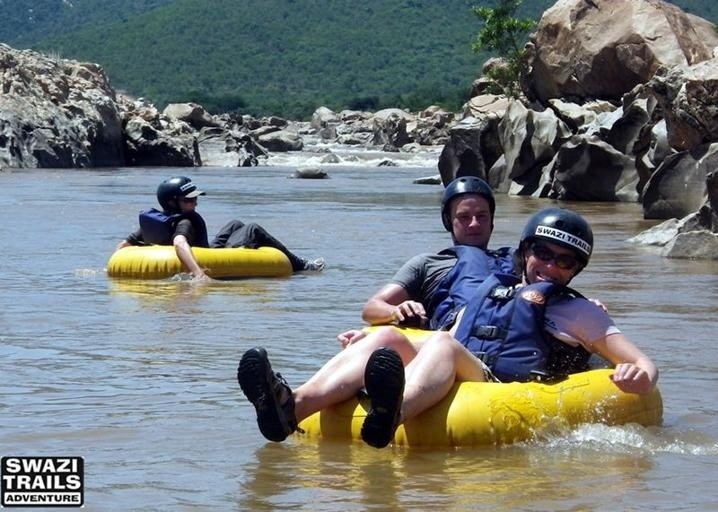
[108,245,293,279]
[293,324,664,447]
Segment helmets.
[441,176,495,231]
[157,176,197,214]
[518,207,593,279]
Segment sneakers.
[298,255,326,272]
[237,347,305,442]
[360,347,405,449]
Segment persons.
[236,207,661,449]
[117,175,326,282]
[360,174,610,326]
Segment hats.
[177,190,206,199]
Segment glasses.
[530,242,581,270]
